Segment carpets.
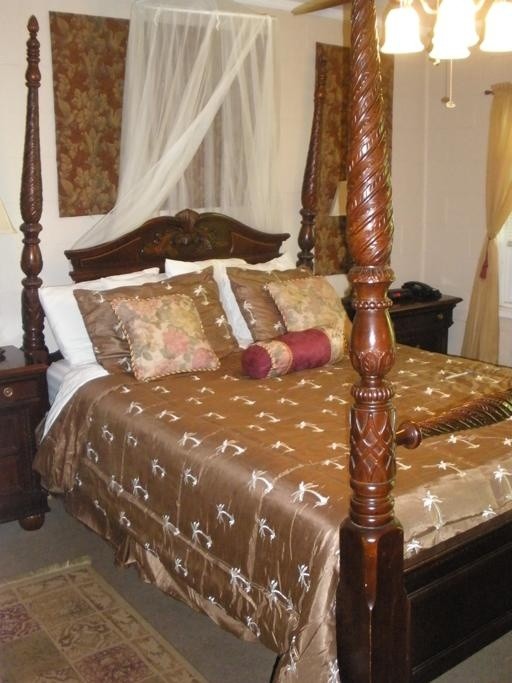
[0,555,209,683]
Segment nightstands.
[1,345,50,531]
[342,295,462,355]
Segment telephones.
[402,281,441,302]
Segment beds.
[46,208,511,683]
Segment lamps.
[382,0,511,110]
